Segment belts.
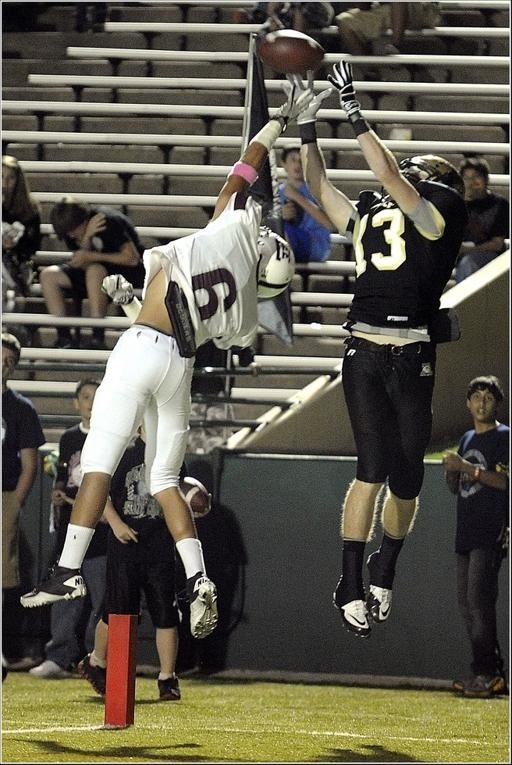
[344,334,435,359]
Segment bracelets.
[475,466,480,478]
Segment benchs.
[2,0,512,431]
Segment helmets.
[396,153,467,195]
[253,218,295,303]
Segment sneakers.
[18,569,89,609]
[331,574,371,638]
[187,577,220,639]
[77,653,107,698]
[13,328,115,348]
[452,671,510,700]
[365,551,395,624]
[8,656,40,670]
[157,673,181,701]
[27,661,72,677]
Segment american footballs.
[230,8,250,23]
[177,476,208,512]
[257,29,325,73]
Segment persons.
[274,145,332,263]
[456,158,510,286]
[234,2,444,55]
[279,61,469,639]
[20,90,315,638]
[1,332,48,669]
[40,202,146,349]
[0,155,42,298]
[73,4,108,31]
[440,376,509,699]
[188,336,261,454]
[78,421,183,702]
[29,378,127,677]
